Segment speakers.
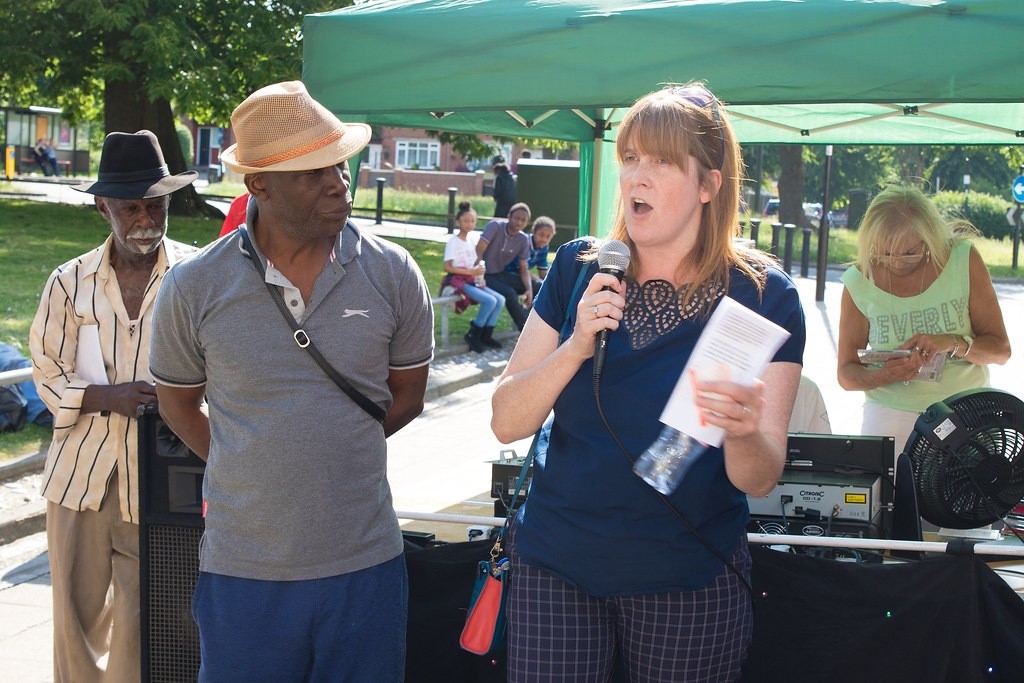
[136,414,208,683]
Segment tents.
[301,0,1024,302]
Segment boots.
[483,326,502,348]
[463,321,485,353]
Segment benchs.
[20,158,71,177]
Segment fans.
[904,387,1024,531]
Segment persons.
[148,81,434,683]
[505,216,556,279]
[33,139,60,176]
[838,186,1011,531]
[476,202,543,333]
[443,202,506,353]
[30,130,209,683]
[491,83,806,683]
[492,154,517,218]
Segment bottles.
[632,425,710,496]
[473,260,486,287]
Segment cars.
[763,199,780,217]
[802,202,833,225]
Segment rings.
[592,306,600,319]
[135,404,145,417]
[740,406,751,418]
[915,367,922,373]
[913,345,920,351]
[920,350,929,356]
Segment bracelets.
[944,333,960,358]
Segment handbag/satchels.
[459,558,514,665]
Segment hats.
[69,130,198,199]
[219,80,372,175]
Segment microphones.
[594,239,632,396]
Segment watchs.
[961,335,973,359]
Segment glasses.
[879,241,925,264]
[670,83,725,176]
